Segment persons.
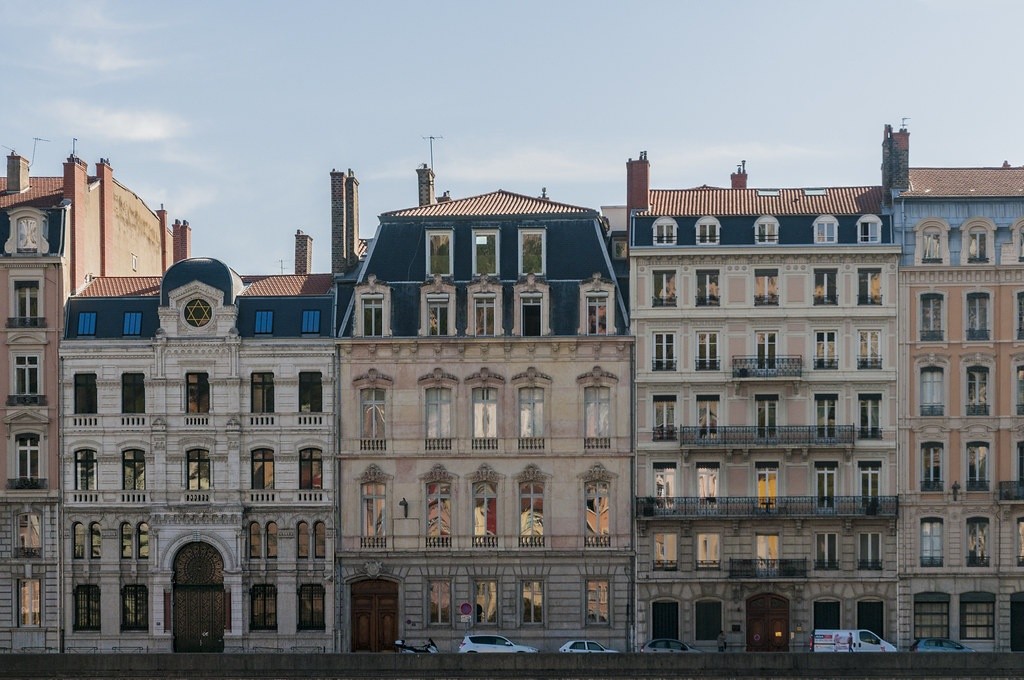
[952,480,961,501]
[716,630,728,653]
[833,633,842,652]
[847,632,854,652]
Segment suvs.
[458,634,543,654]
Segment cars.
[910,637,976,653]
[640,638,700,651]
[561,639,619,654]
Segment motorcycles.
[393,637,441,654]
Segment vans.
[810,629,897,653]
